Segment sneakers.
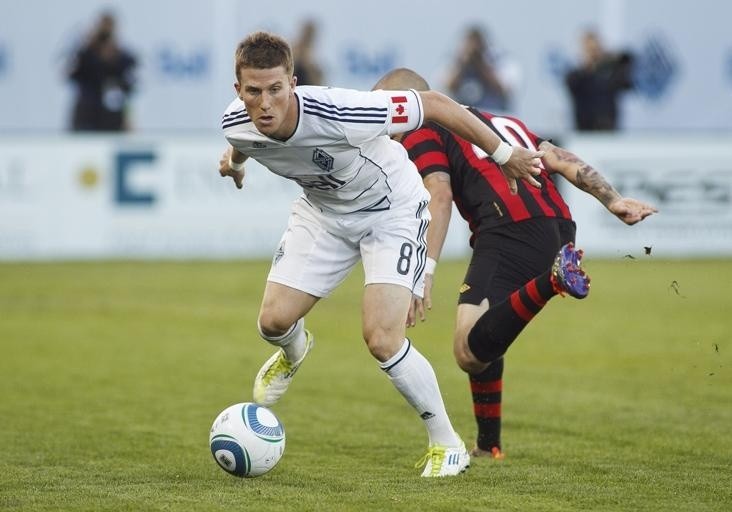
[551,241,589,299]
[422,442,470,477]
[471,446,505,461]
[253,329,314,405]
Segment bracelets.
[489,140,514,166]
[424,257,437,273]
[228,157,247,171]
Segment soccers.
[209,403,284,476]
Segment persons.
[563,33,635,131]
[292,20,322,88]
[64,14,138,131]
[216,30,545,477]
[441,29,518,114]
[369,65,659,463]
